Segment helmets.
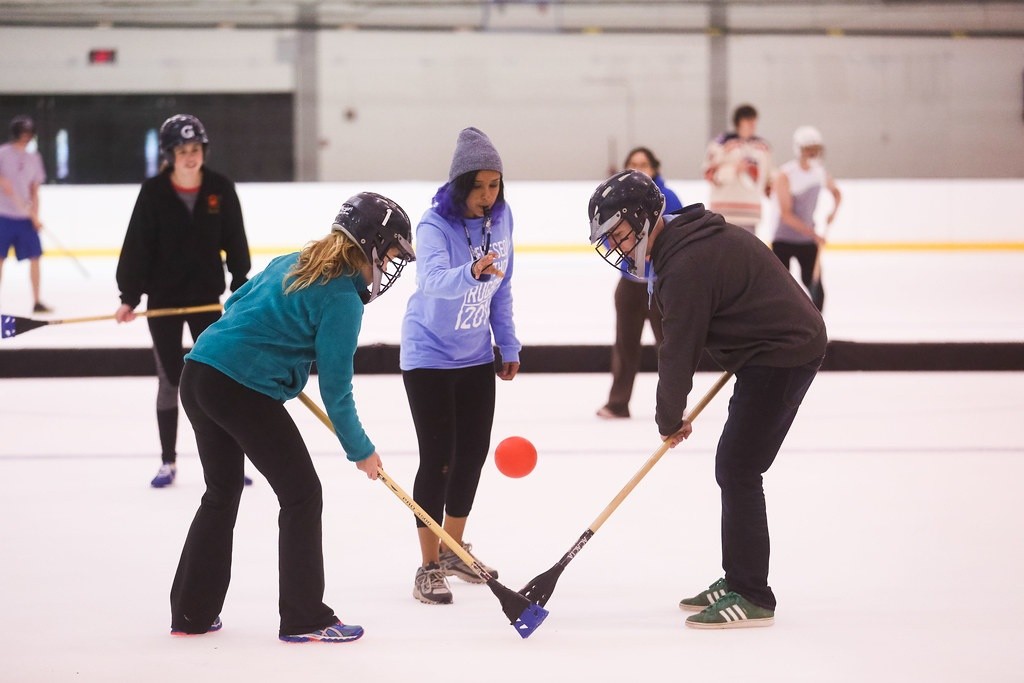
[792,126,825,158]
[159,114,208,154]
[331,192,416,305]
[588,169,666,279]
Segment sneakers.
[686,591,776,628]
[438,541,499,584]
[413,560,453,605]
[151,466,176,488]
[244,476,252,485]
[681,579,730,610]
[171,617,222,635]
[279,620,364,643]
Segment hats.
[448,127,503,183]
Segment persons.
[0,116,54,313]
[588,170,827,629]
[117,114,253,489]
[171,192,416,642]
[595,148,683,418]
[400,126,522,604]
[774,125,842,308]
[702,105,774,233]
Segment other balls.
[494,436,536,480]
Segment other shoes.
[33,303,49,311]
